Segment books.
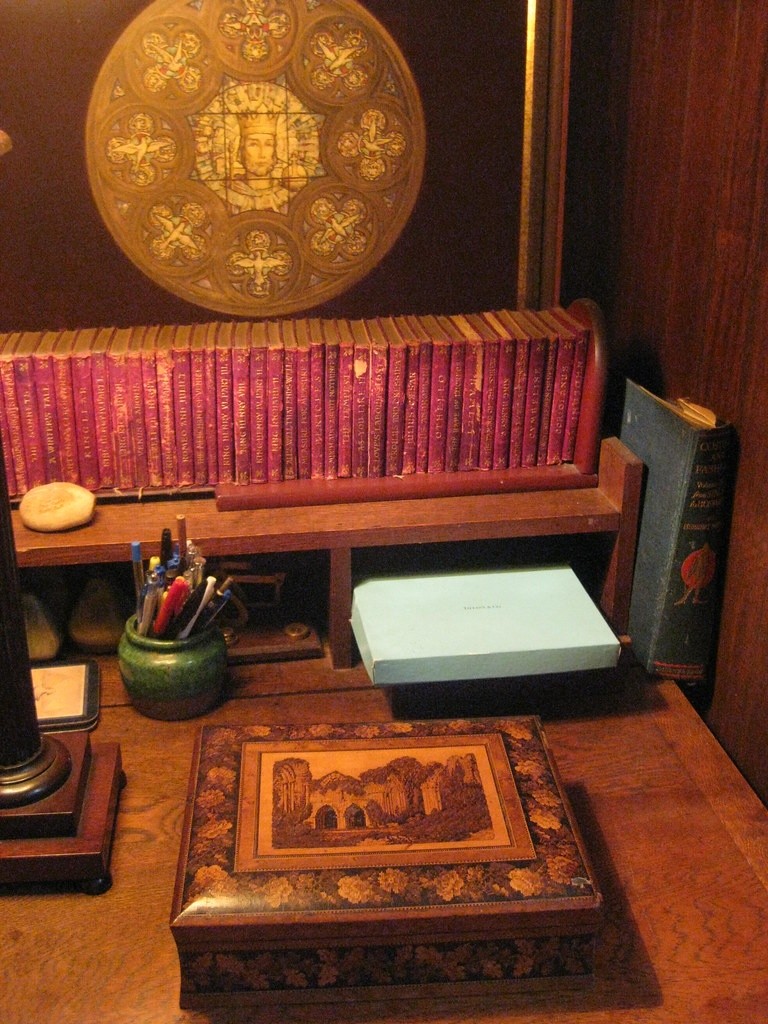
[618,371,738,682]
[0,304,591,500]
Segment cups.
[116,612,233,721]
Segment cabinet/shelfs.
[8,436,649,672]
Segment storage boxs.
[350,565,621,719]
[170,715,604,1010]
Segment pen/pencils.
[130,512,234,641]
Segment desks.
[1,667,767,1024]
